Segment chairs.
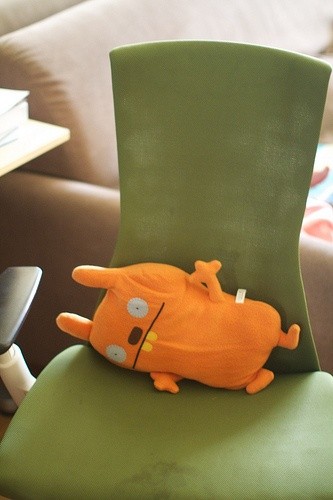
[1,39,333,500]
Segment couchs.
[0,1,333,372]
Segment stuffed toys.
[56,258,303,399]
